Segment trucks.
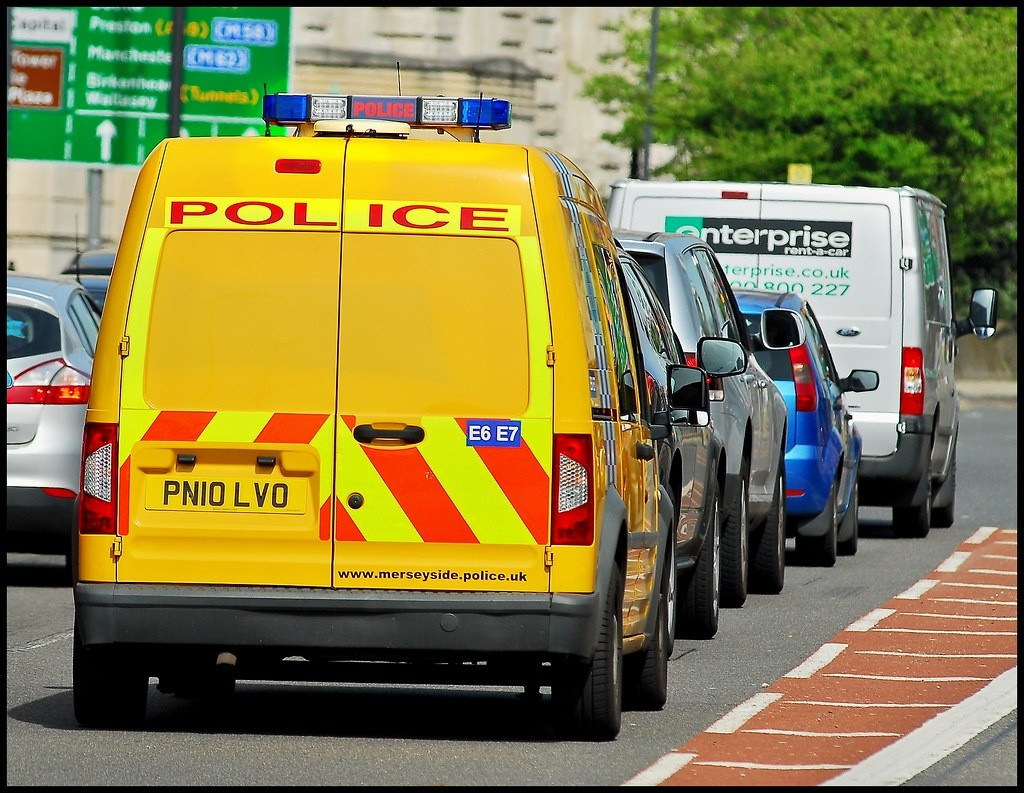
[609,177,998,539]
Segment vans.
[70,93,709,742]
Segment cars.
[6,273,102,588]
[71,275,111,309]
[61,249,117,276]
[613,227,806,610]
[733,287,880,567]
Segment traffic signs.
[8,6,295,169]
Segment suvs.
[613,237,748,659]
